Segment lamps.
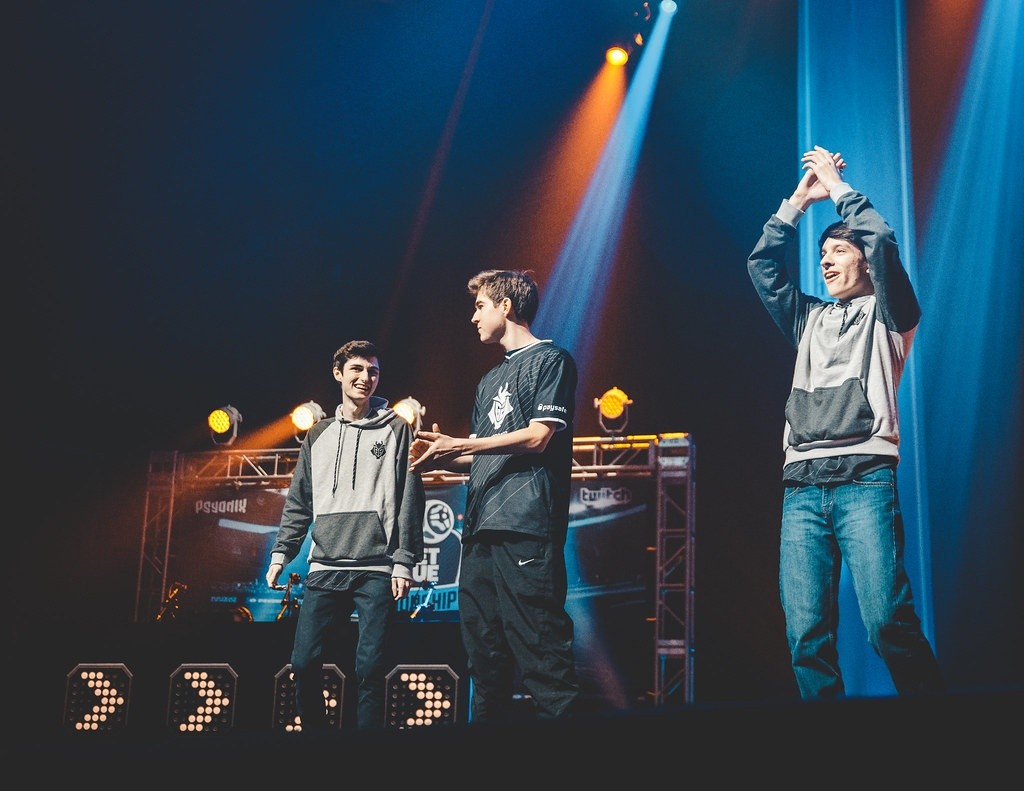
[392,396,426,437]
[288,401,327,443]
[207,403,243,449]
[592,386,634,436]
[605,32,635,64]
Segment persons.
[266,340,426,734]
[408,270,579,734]
[747,146,943,715]
